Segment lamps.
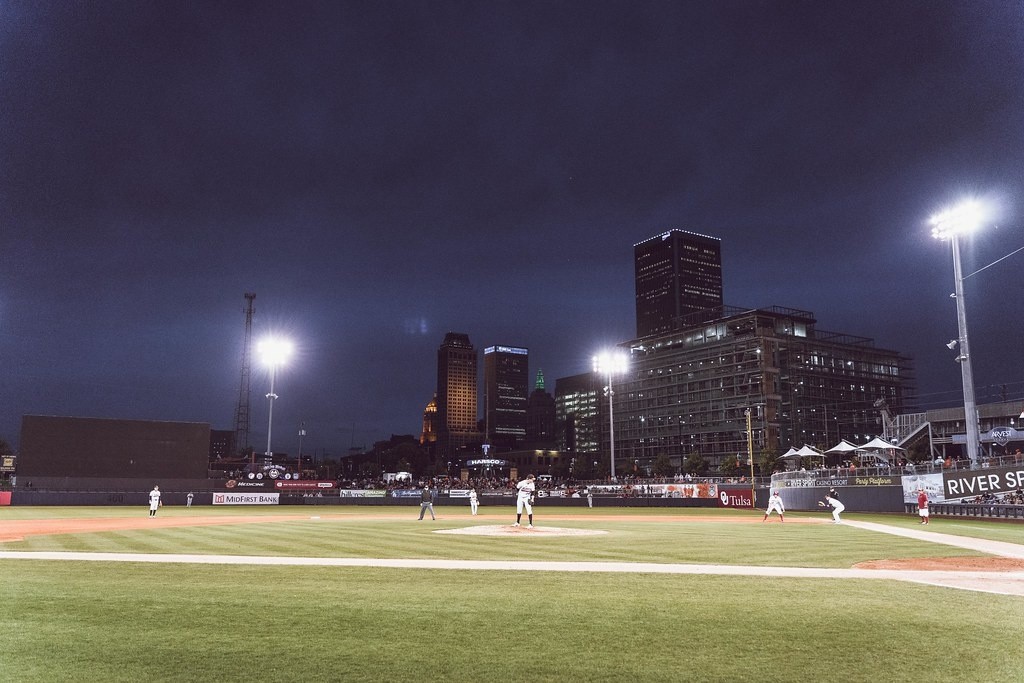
[1010,416,1014,425]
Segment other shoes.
[921,521,928,525]
[511,522,520,527]
[525,524,533,529]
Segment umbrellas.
[822,440,859,459]
[859,437,905,463]
[775,445,826,469]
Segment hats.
[773,491,778,495]
[527,474,536,480]
[918,489,923,492]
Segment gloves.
[430,503,432,506]
[420,503,422,506]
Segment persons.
[819,494,845,524]
[785,459,913,478]
[418,486,435,520]
[469,488,479,516]
[512,474,536,528]
[27,480,32,487]
[830,487,839,521]
[297,471,322,497]
[850,463,855,475]
[338,473,747,498]
[186,491,193,507]
[587,492,593,508]
[762,490,785,522]
[221,469,240,480]
[934,449,1024,510]
[916,488,929,525]
[149,485,162,518]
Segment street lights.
[295,421,308,472]
[260,339,287,469]
[594,350,627,484]
[931,209,982,464]
